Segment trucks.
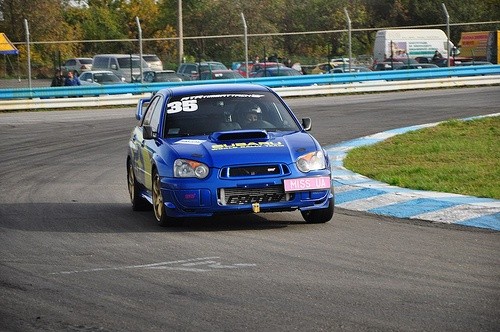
[373,29,460,63]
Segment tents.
[0,33,20,82]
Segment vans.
[91,53,151,83]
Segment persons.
[51,70,83,99]
[284,58,304,75]
[253,55,269,64]
[432,50,444,67]
[240,103,264,132]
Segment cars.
[131,54,493,83]
[57,58,94,75]
[77,71,123,97]
[126,84,335,225]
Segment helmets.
[244,104,263,123]
